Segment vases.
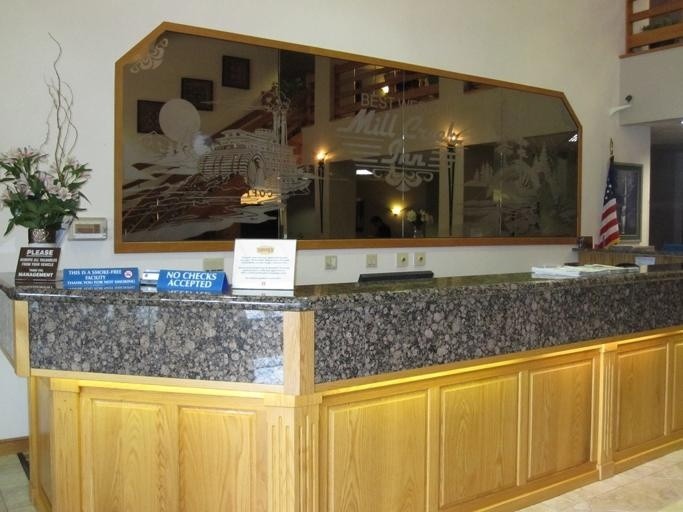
[26,226,52,245]
[410,225,425,239]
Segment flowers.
[0,143,90,232]
[402,208,433,226]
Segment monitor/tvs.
[359,270,433,282]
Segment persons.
[367,216,392,238]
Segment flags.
[594,155,620,249]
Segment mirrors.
[112,19,581,254]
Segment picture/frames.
[178,75,213,112]
[605,160,644,244]
[135,100,168,135]
[221,55,251,89]
